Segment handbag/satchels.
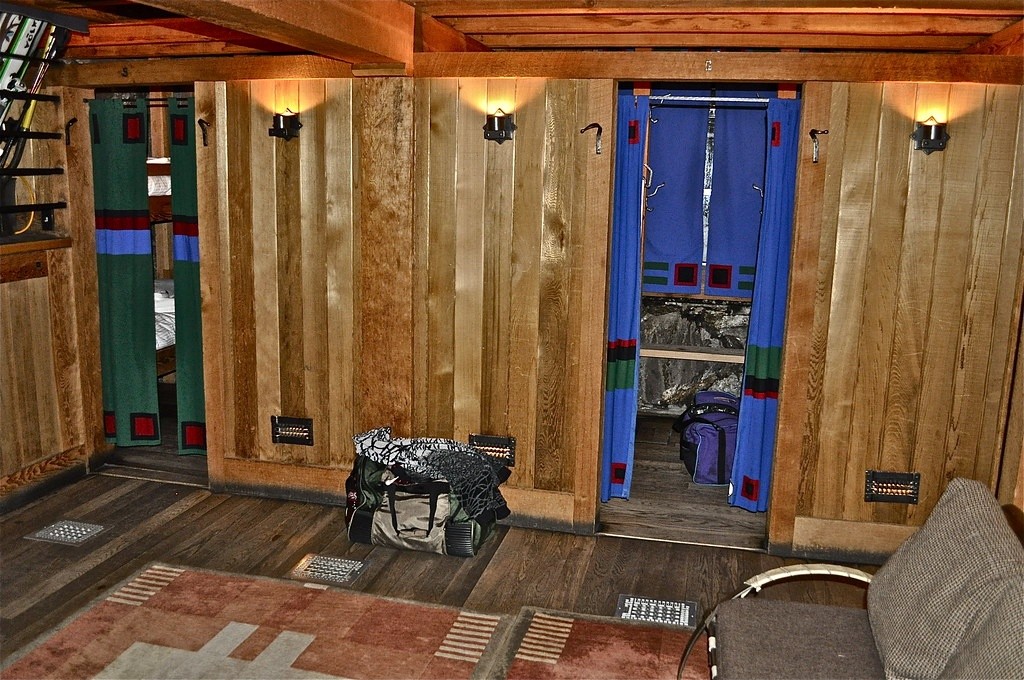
[672,392,740,487]
[345,452,496,558]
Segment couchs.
[676,477,1024,680]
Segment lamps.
[482,108,518,145]
[268,108,303,141]
[909,116,950,156]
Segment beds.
[154,278,175,380]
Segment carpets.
[0,560,709,680]
